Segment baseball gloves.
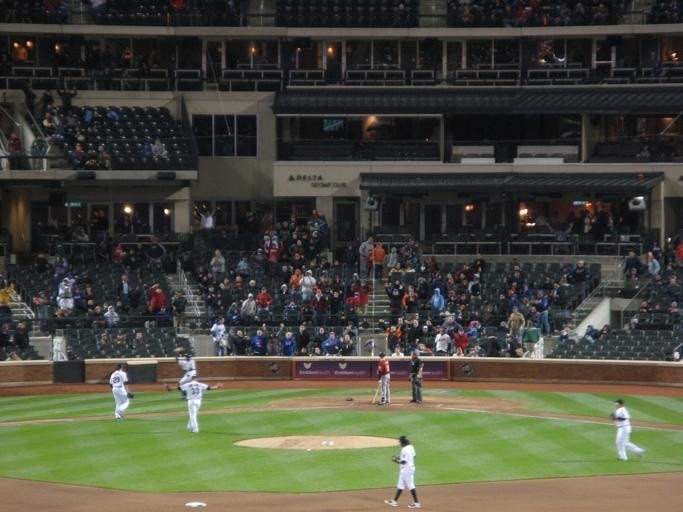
[126,392,134,398]
[165,383,170,392]
[391,454,400,462]
[415,375,422,387]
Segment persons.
[166,375,224,434]
[384,435,422,509]
[107,363,134,419]
[0,1,189,172]
[176,351,198,398]
[449,1,683,29]
[373,38,557,72]
[406,350,425,404]
[375,350,391,406]
[609,396,645,462]
[0,204,683,362]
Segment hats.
[399,435,410,442]
[614,399,625,405]
[295,268,328,296]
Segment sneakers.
[114,409,125,421]
[384,499,398,507]
[616,448,645,462]
[408,502,423,509]
[409,398,423,404]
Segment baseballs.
[307,449,310,452]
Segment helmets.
[184,353,193,358]
[378,351,386,356]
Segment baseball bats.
[371,383,382,403]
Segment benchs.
[2,0,201,170]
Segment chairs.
[275,0,424,27]
